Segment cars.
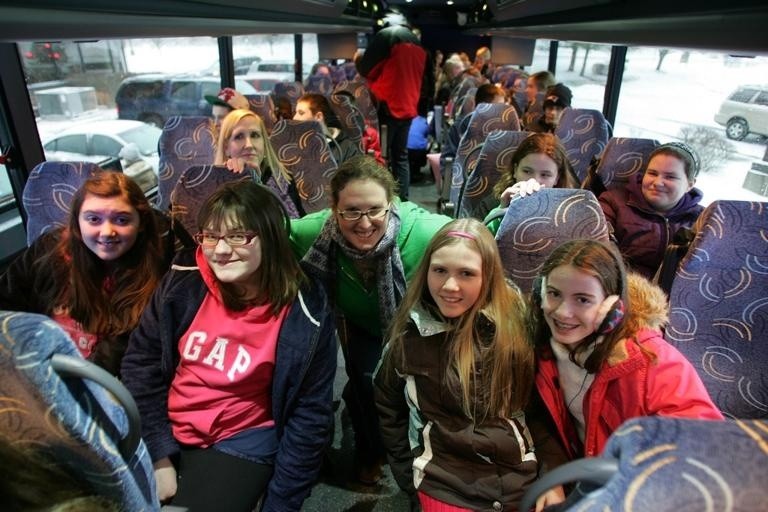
[36,115,163,176]
[200,54,311,93]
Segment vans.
[113,73,260,128]
[715,80,767,144]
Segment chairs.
[2,309,162,511]
[658,198,765,420]
[492,187,612,296]
[431,69,660,221]
[242,64,389,217]
[167,164,240,241]
[521,417,765,509]
[21,160,102,248]
[156,115,223,215]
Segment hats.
[543,83,572,109]
[205,87,251,111]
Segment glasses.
[337,207,389,221]
[192,230,258,247]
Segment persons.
[372,217,571,512]
[526,238,726,498]
[281,154,547,485]
[120,178,338,512]
[204,5,708,298]
[0,171,186,381]
